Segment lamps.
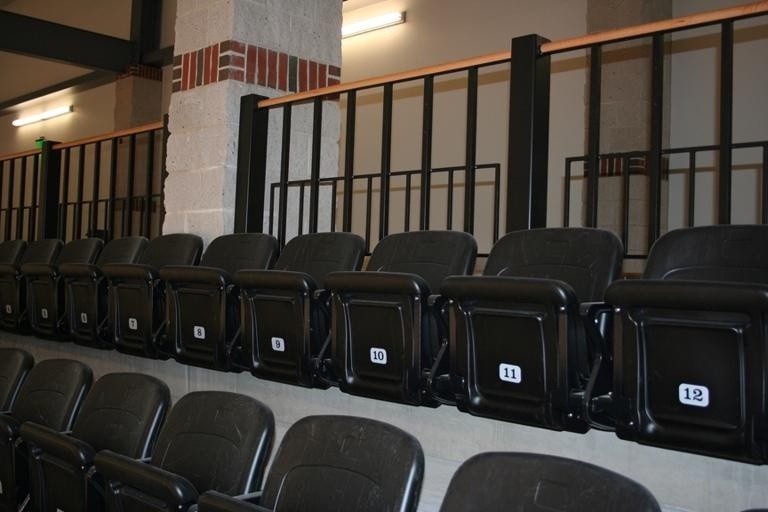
[342,10,406,40]
[12,105,73,127]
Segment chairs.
[151,233,279,374]
[577,223,767,468]
[1,359,92,512]
[0,348,34,416]
[312,230,478,409]
[225,232,365,391]
[426,226,623,433]
[188,414,425,512]
[438,451,661,512]
[1,233,204,362]
[86,391,275,512]
[11,373,171,512]
